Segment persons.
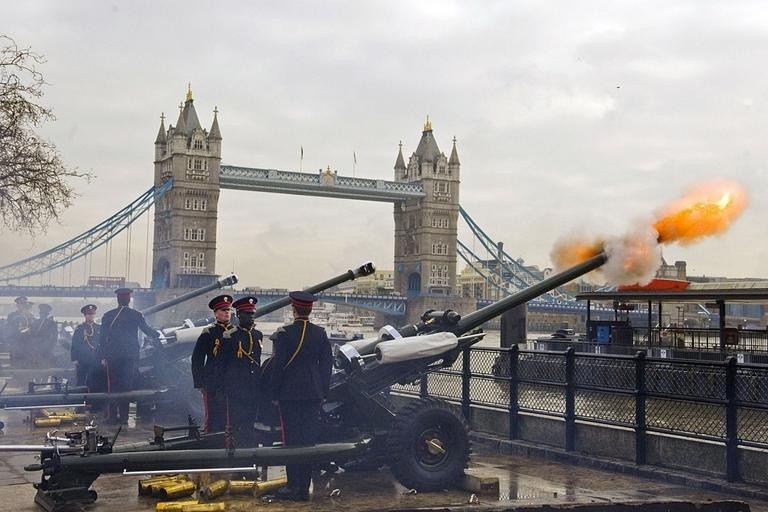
[8,300,35,370]
[4,295,28,364]
[98,288,163,426]
[69,303,101,416]
[189,295,233,436]
[212,296,264,481]
[260,289,334,503]
[27,303,61,391]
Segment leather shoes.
[273,485,309,501]
[101,418,127,424]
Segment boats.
[491,285,766,411]
[331,328,367,342]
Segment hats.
[289,291,318,308]
[208,295,233,312]
[232,297,257,312]
[115,288,133,297]
[14,296,52,311]
[81,304,97,314]
